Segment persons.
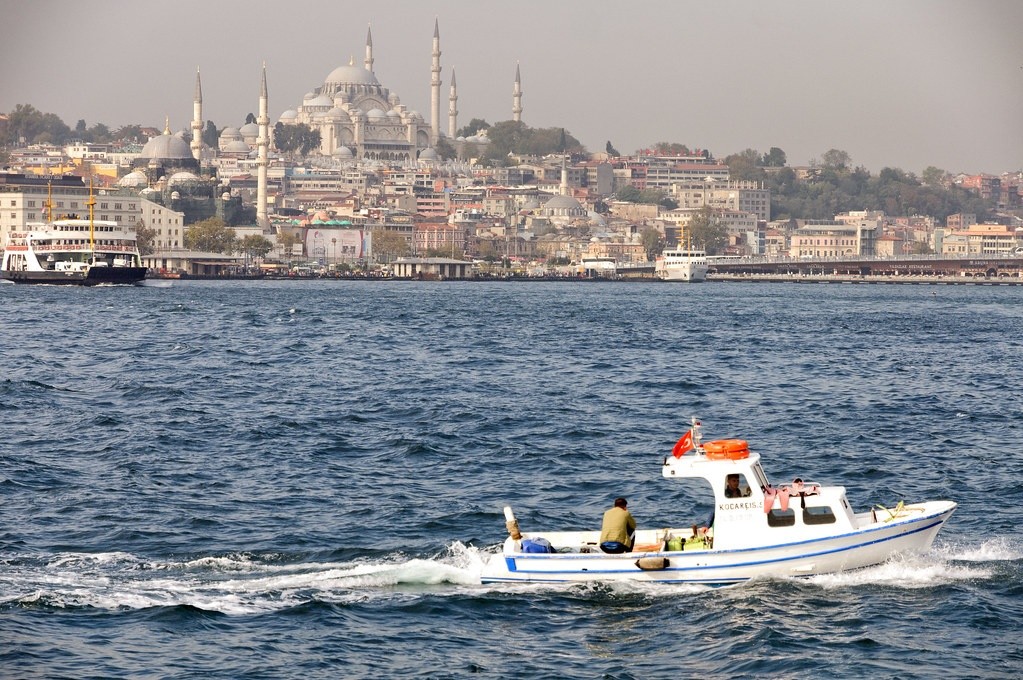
[146,266,177,274]
[599,497,637,554]
[54,212,80,220]
[479,270,587,278]
[246,266,394,277]
[701,474,751,534]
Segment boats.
[0,175,148,286]
[480,414,958,589]
[654,223,707,282]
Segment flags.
[672,429,696,460]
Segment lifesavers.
[702,437,750,460]
